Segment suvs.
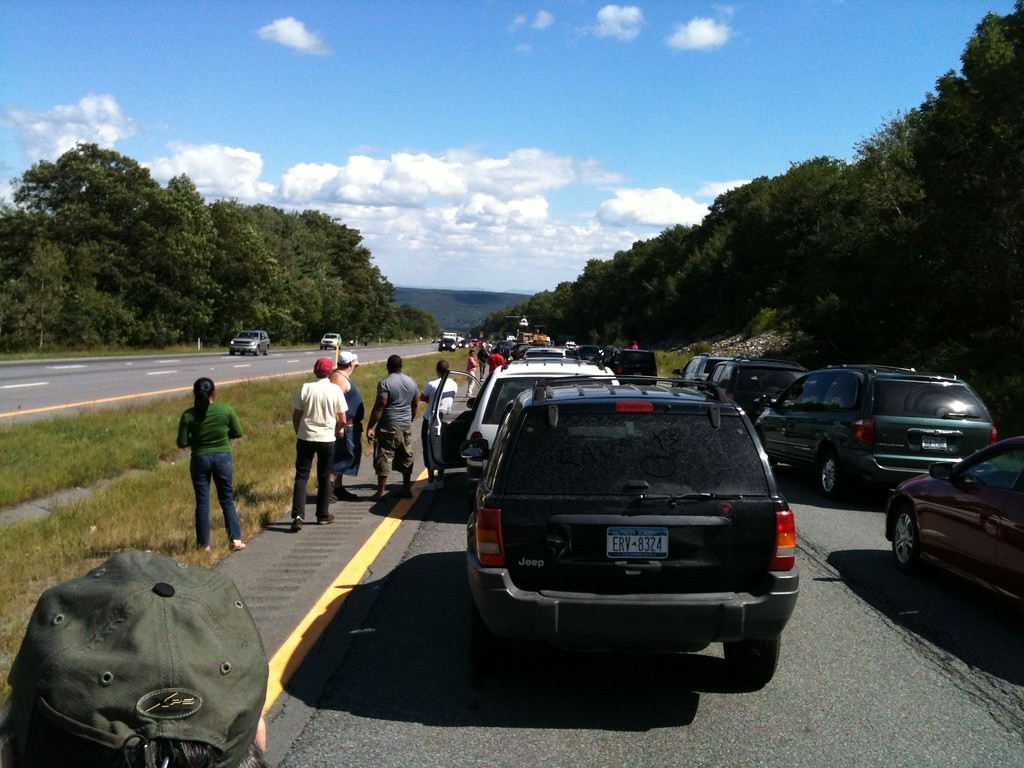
[751,364,998,505]
[603,349,658,387]
[705,359,811,424]
[229,330,270,356]
[466,375,800,685]
[426,357,621,501]
[671,352,750,392]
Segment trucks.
[438,332,457,352]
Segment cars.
[432,335,479,348]
[498,330,605,363]
[884,435,1024,606]
[319,333,343,350]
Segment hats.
[6,548,269,768]
[314,358,333,377]
[339,351,354,362]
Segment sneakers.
[333,485,357,501]
[327,481,338,503]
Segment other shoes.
[390,489,412,499]
[317,515,334,524]
[434,479,443,488]
[416,481,436,490]
[198,545,210,551]
[367,492,386,502]
[291,519,302,530]
[229,540,246,549]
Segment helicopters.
[505,313,544,329]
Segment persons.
[175,376,249,551]
[415,359,458,491]
[1,549,271,768]
[465,350,480,398]
[632,340,638,350]
[366,355,421,502]
[328,351,365,505]
[477,339,539,382]
[290,358,348,533]
